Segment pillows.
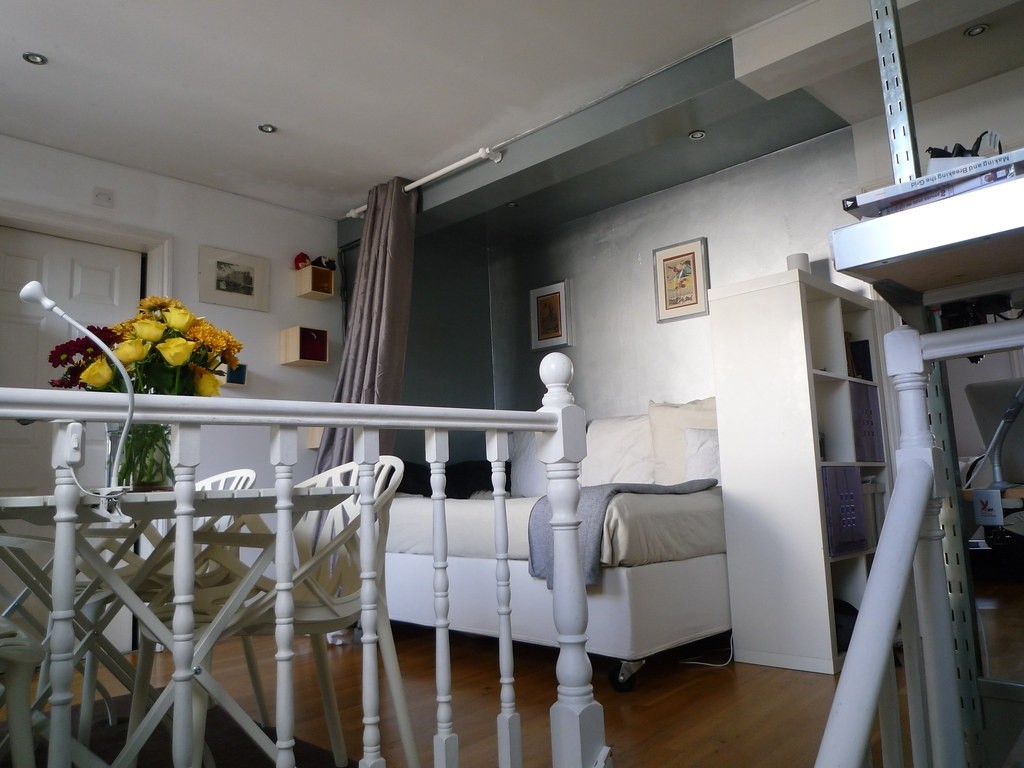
[649,396,717,486]
[511,428,582,497]
[686,428,722,485]
[582,414,654,484]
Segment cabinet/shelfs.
[707,268,894,674]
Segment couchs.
[353,395,733,693]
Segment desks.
[0,485,359,768]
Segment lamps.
[18,280,135,524]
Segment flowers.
[47,296,243,428]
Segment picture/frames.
[199,244,271,313]
[652,237,708,324]
[526,278,573,353]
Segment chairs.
[129,454,405,768]
[37,467,272,752]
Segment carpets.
[0,686,360,768]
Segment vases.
[107,429,171,487]
